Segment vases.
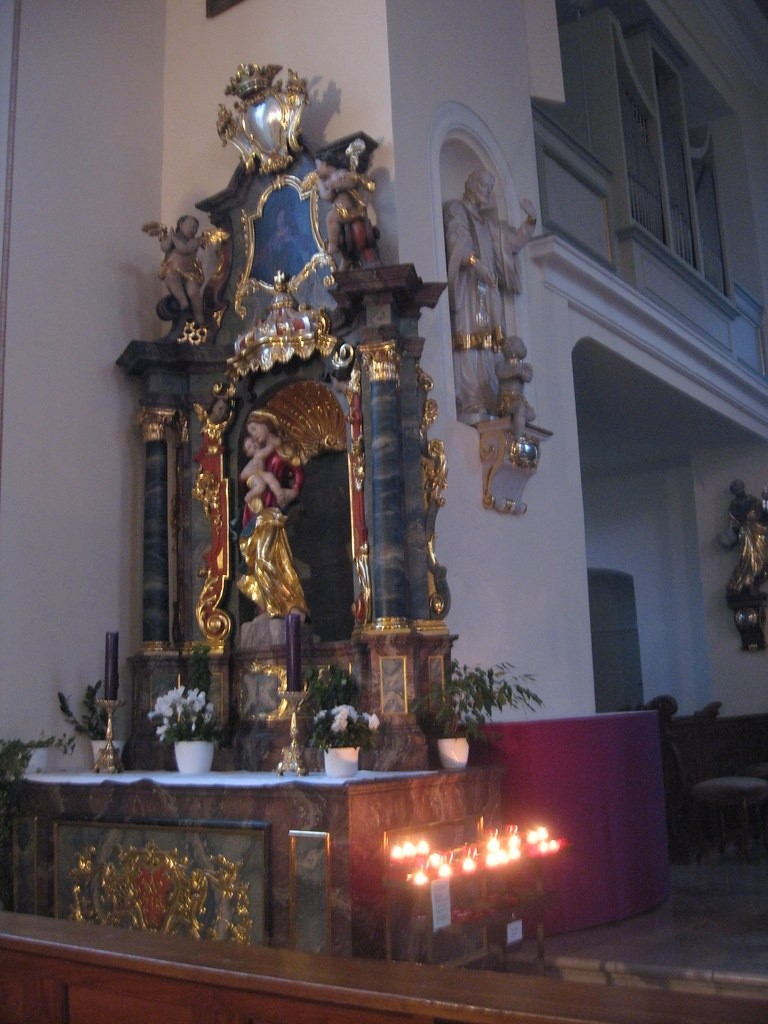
[174,741,214,775]
[324,747,359,778]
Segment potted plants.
[408,659,545,770]
[1,731,75,774]
[57,679,126,774]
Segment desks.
[445,708,671,943]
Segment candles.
[286,613,302,692]
[391,822,560,884]
[104,630,119,701]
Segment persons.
[446,168,537,419]
[314,150,380,265]
[159,214,205,327]
[717,479,768,596]
[239,410,310,622]
[495,335,539,442]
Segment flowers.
[307,668,377,747]
[148,687,222,742]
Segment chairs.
[647,695,768,862]
